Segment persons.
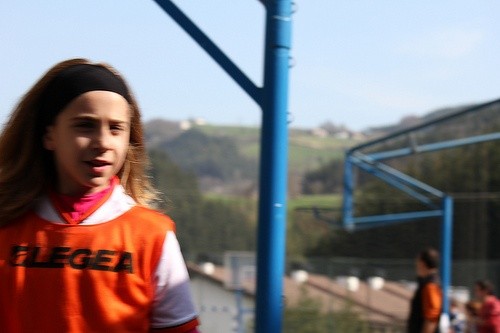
[407,247,443,333]
[475,279,500,333]
[0,57,202,333]
